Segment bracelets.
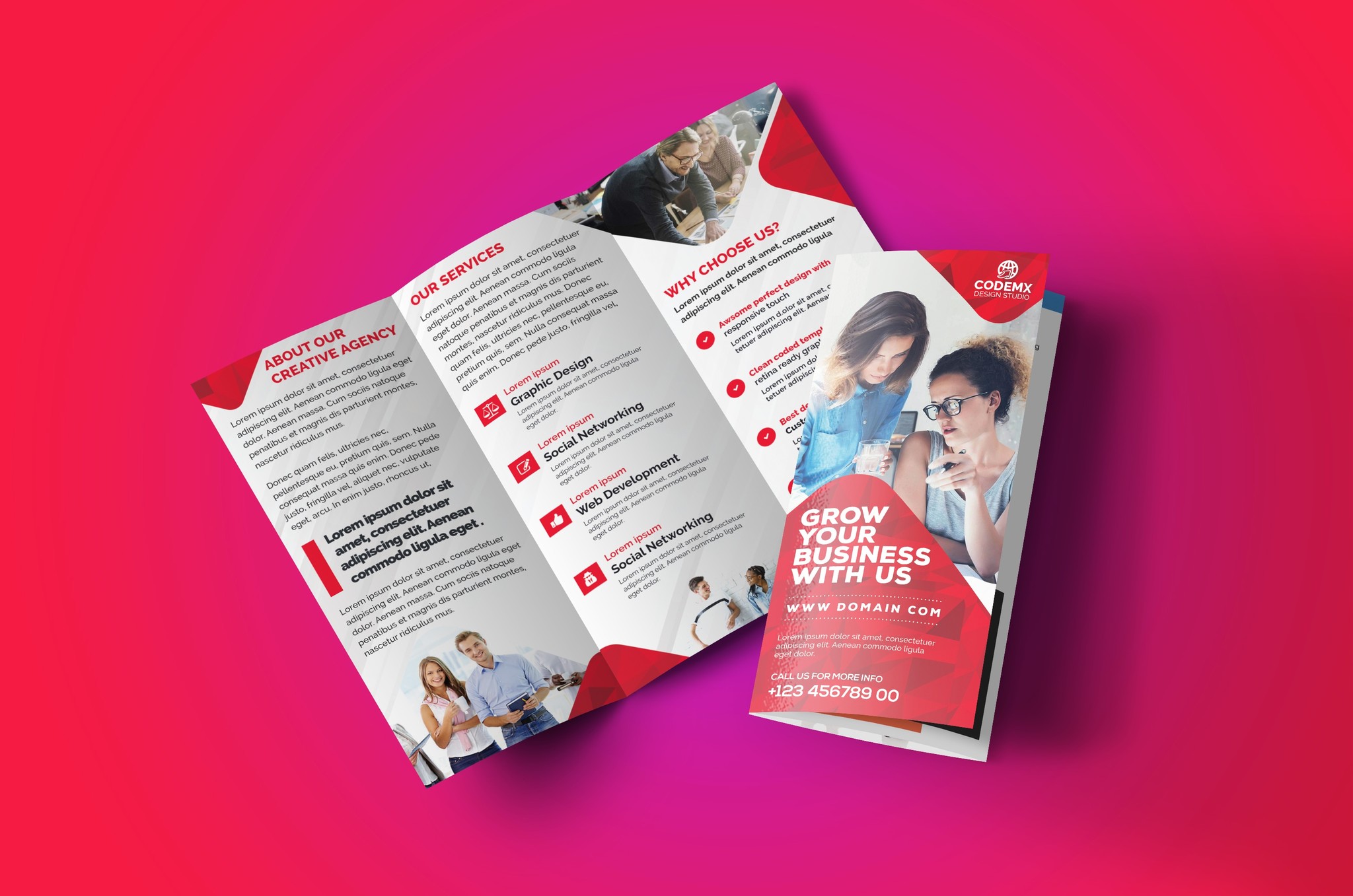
[732,177,742,183]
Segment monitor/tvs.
[890,410,918,445]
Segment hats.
[732,111,754,125]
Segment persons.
[390,631,588,790]
[688,576,743,649]
[745,566,775,618]
[790,290,930,498]
[891,337,1027,578]
[602,116,745,246]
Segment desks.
[677,163,750,244]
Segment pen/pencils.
[522,698,527,701]
[714,191,729,196]
[686,219,705,231]
[939,449,966,475]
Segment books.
[557,680,576,691]
[506,692,536,722]
[408,732,431,758]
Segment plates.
[560,676,574,682]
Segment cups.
[565,202,575,209]
[559,671,570,680]
[855,439,891,478]
[453,696,470,712]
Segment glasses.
[745,575,759,581]
[922,392,989,420]
[669,148,704,164]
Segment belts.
[499,707,547,729]
